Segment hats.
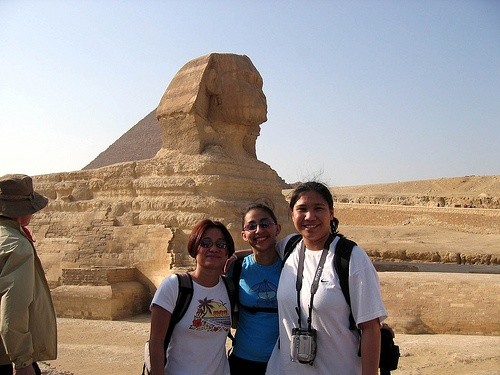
[0,174,48,217]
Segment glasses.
[199,238,228,249]
[244,221,275,230]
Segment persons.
[142,218,239,375]
[223,204,283,375]
[224,181,388,375]
[0,173,57,375]
